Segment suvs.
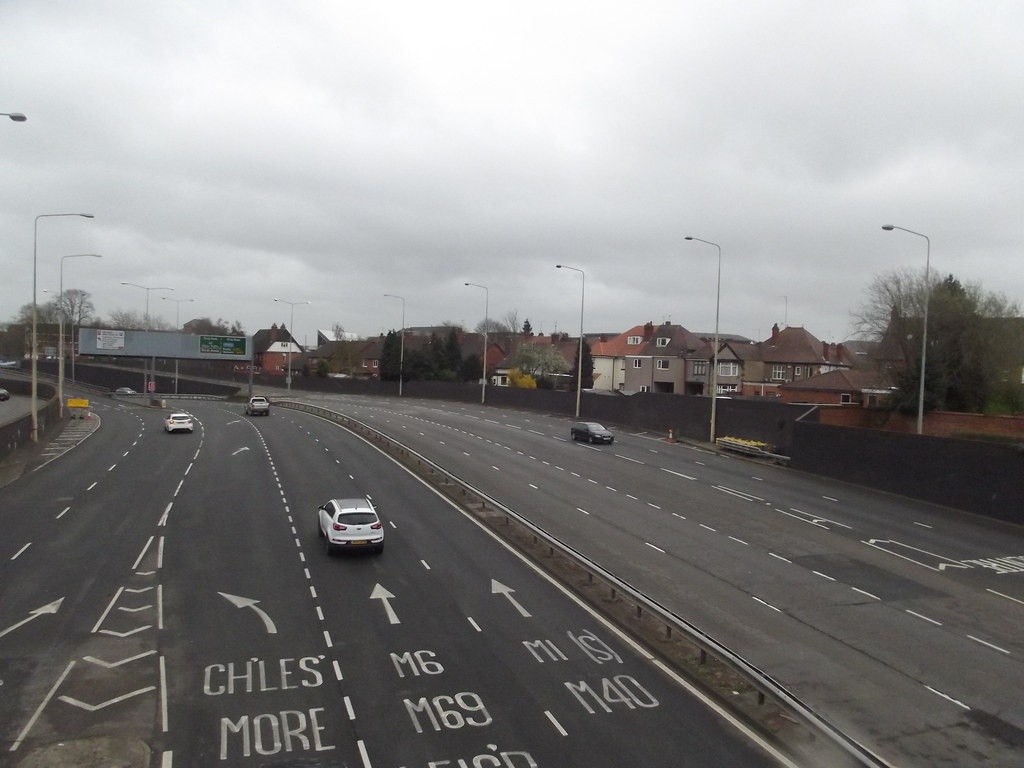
[317,497,385,554]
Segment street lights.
[161,297,194,394]
[685,236,721,442]
[41,288,66,380]
[556,264,585,417]
[273,297,311,394]
[57,253,102,420]
[882,224,930,436]
[464,282,488,406]
[30,213,95,444]
[121,282,175,406]
[383,294,406,398]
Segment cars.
[570,422,614,445]
[162,413,193,433]
[115,387,137,397]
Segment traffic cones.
[667,429,677,443]
[85,411,91,421]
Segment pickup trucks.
[245,396,271,416]
